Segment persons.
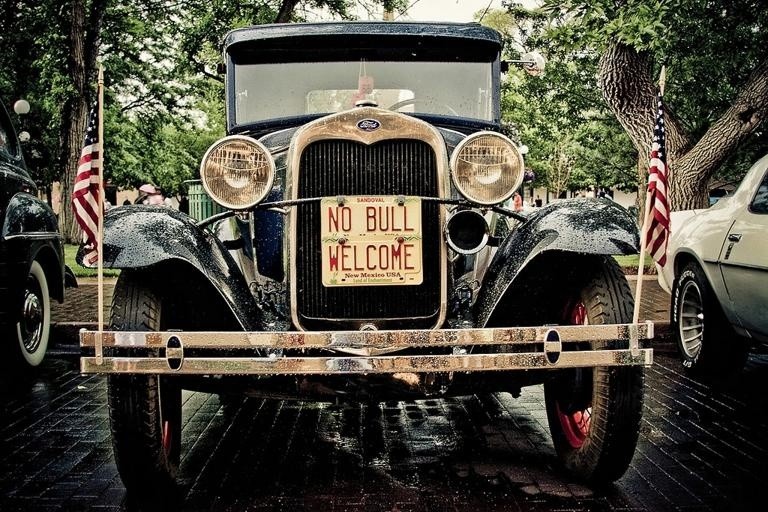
[513,191,542,212]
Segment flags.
[644,86,671,271]
[72,86,104,270]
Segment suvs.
[0,97,82,375]
[78,14,658,503]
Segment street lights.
[12,99,32,165]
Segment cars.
[654,152,767,378]
